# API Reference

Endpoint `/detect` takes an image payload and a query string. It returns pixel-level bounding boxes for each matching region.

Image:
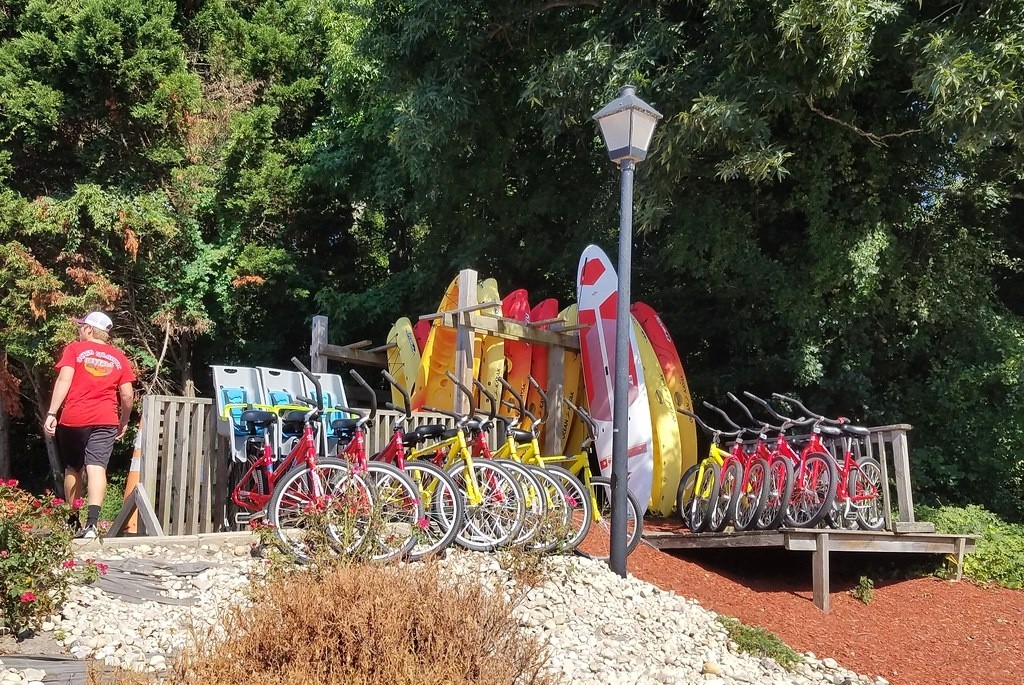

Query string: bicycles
[380,369,465,563]
[540,396,644,565]
[676,389,891,531]
[416,379,549,547]
[325,367,424,569]
[227,356,382,567]
[501,375,593,556]
[389,371,526,554]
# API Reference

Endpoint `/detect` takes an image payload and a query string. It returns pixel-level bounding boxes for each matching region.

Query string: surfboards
[385,267,591,475]
[574,244,654,520]
[630,301,698,518]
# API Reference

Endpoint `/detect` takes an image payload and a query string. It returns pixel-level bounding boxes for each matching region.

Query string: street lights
[589,83,665,580]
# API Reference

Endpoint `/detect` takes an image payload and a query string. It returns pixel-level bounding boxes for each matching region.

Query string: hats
[72,311,114,333]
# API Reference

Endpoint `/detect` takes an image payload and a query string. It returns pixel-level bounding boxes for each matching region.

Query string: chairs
[208,365,279,461]
[298,372,352,458]
[255,366,322,460]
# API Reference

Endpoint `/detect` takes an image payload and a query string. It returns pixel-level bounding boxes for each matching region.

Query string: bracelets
[47,413,56,416]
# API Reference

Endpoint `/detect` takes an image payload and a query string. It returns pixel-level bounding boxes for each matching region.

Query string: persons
[43,311,136,538]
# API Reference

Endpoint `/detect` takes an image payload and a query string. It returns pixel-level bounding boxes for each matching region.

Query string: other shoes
[81,527,97,538]
[68,524,81,538]
